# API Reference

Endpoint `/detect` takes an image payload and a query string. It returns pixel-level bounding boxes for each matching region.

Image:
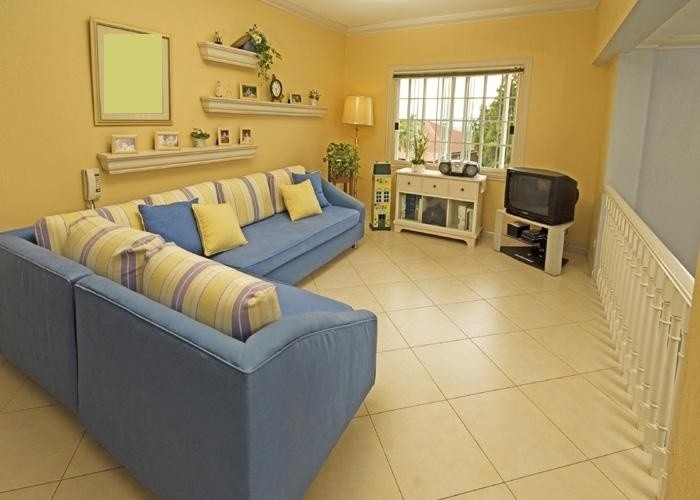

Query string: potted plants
[410,131,430,173]
[323,142,360,178]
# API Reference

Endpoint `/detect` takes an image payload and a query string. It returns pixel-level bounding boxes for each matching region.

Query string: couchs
[2,165,366,417]
[70,267,377,500]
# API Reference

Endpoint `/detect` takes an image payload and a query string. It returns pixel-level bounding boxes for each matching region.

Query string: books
[400,194,418,221]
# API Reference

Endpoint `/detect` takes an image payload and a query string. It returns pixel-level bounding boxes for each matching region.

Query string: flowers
[191,128,210,139]
[309,88,321,99]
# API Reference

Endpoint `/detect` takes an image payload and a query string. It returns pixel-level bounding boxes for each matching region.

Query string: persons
[242,131,251,142]
[246,88,254,97]
[222,131,228,143]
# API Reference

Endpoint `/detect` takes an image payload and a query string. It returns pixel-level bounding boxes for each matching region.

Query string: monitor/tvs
[504,167,579,225]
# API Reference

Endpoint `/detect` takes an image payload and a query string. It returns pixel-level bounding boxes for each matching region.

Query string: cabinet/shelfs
[491,208,575,277]
[327,172,355,199]
[97,39,329,177]
[393,167,487,248]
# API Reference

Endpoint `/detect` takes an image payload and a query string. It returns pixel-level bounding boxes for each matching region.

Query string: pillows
[138,198,204,257]
[278,179,322,222]
[291,170,330,209]
[191,200,248,257]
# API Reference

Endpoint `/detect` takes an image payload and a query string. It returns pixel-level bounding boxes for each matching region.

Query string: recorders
[439,160,480,177]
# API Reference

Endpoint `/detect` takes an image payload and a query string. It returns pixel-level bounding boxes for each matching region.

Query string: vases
[310,98,320,105]
[191,138,209,147]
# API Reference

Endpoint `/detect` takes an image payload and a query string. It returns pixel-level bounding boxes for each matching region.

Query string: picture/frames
[154,131,182,151]
[239,83,260,100]
[89,15,174,126]
[240,126,255,145]
[217,128,231,145]
[290,93,303,104]
[111,135,138,155]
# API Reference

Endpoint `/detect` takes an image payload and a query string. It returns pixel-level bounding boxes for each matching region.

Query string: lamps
[341,96,374,156]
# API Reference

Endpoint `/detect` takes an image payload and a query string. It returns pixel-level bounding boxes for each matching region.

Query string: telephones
[82,168,102,201]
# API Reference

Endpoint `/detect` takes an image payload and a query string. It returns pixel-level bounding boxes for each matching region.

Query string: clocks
[270,74,284,103]
